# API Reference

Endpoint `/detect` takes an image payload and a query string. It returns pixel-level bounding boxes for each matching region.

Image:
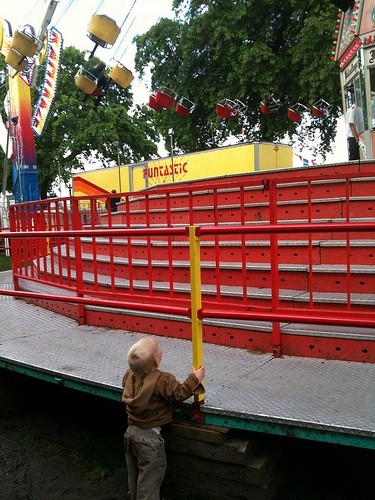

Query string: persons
[122,338,205,500]
[346,90,364,160]
[105,190,121,212]
